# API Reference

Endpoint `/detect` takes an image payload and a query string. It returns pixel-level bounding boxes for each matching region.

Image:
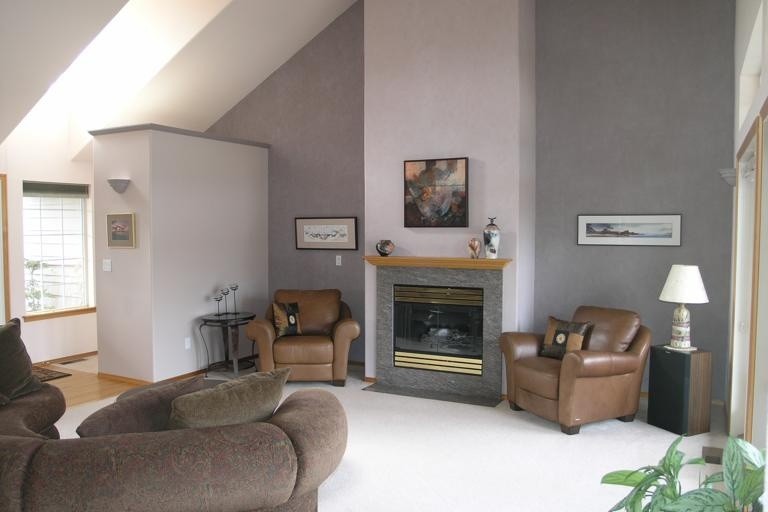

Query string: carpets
[32,364,72,383]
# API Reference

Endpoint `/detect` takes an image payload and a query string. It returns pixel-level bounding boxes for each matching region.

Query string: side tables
[201,311,257,373]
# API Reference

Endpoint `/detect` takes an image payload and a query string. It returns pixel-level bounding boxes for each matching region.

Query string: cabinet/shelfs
[648,342,714,437]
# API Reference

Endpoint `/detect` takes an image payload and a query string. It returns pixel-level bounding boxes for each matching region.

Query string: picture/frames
[576,213,682,247]
[295,216,358,250]
[105,212,137,250]
[403,158,469,228]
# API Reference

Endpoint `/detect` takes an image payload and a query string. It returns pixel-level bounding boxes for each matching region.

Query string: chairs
[245,300,361,387]
[0,383,349,512]
[499,305,653,435]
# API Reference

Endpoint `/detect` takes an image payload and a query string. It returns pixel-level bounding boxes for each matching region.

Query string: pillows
[274,289,342,336]
[169,365,293,429]
[539,317,592,360]
[271,301,303,339]
[0,318,41,409]
[75,373,206,437]
[572,306,641,352]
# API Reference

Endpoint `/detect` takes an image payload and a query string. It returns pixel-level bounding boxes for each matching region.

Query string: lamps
[658,263,710,353]
[718,168,736,187]
[211,282,240,317]
[107,178,130,194]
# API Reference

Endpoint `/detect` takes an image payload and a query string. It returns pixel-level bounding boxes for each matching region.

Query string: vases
[375,240,394,257]
[483,217,501,260]
[468,238,481,259]
